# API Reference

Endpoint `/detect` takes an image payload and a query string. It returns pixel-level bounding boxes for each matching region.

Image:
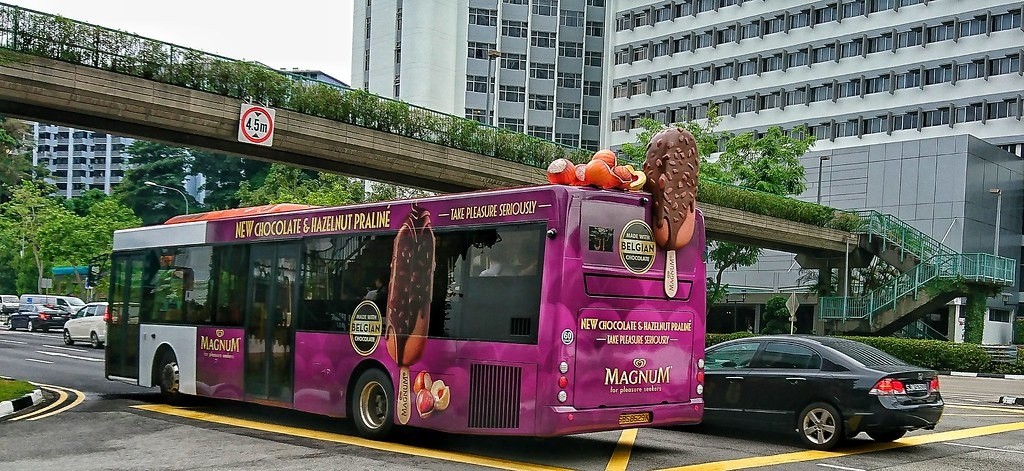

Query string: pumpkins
[546,149,633,190]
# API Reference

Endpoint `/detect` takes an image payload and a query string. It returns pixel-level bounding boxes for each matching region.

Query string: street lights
[818,156,830,205]
[144,181,189,214]
[486,49,500,126]
[989,188,1003,256]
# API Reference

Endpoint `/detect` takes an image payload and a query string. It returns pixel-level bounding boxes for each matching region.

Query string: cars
[7,303,70,332]
[677,334,945,449]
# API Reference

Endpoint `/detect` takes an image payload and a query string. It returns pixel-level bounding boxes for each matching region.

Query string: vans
[19,294,86,316]
[0,294,20,312]
[62,302,142,348]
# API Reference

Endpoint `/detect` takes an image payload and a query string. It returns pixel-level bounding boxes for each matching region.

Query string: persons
[343,262,385,302]
[479,236,538,276]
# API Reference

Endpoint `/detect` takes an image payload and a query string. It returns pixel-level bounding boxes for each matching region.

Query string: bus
[87,126,707,441]
